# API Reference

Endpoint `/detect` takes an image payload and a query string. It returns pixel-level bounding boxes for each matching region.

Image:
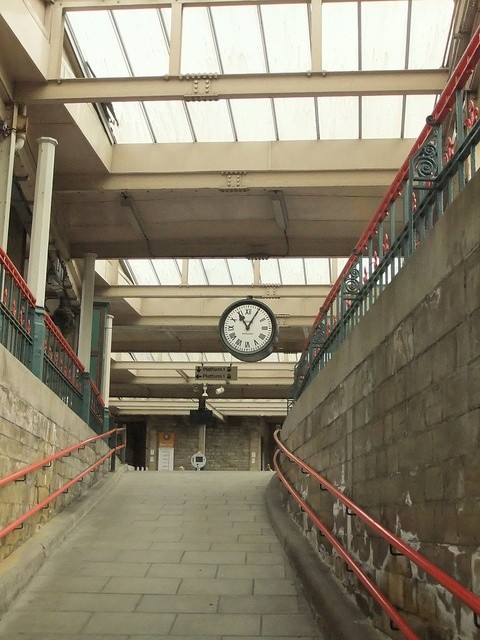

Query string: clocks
[216,298,279,362]
